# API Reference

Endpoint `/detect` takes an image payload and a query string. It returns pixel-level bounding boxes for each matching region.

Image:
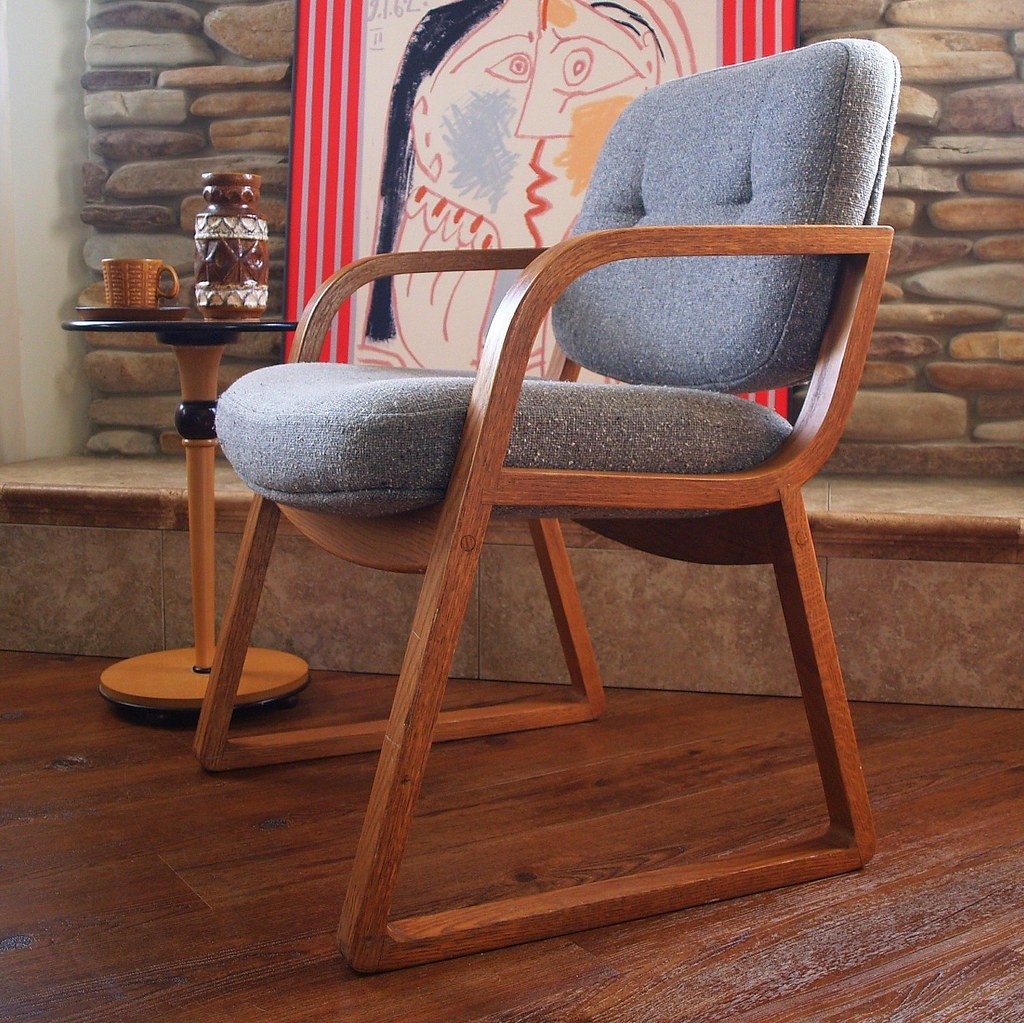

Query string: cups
[102,257,181,307]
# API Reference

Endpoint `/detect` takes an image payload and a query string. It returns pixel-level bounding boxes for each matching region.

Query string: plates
[74,305,192,321]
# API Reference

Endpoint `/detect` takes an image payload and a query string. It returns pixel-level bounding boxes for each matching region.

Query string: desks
[62,319,312,728]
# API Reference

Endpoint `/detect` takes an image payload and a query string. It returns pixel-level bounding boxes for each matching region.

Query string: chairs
[189,36,901,975]
